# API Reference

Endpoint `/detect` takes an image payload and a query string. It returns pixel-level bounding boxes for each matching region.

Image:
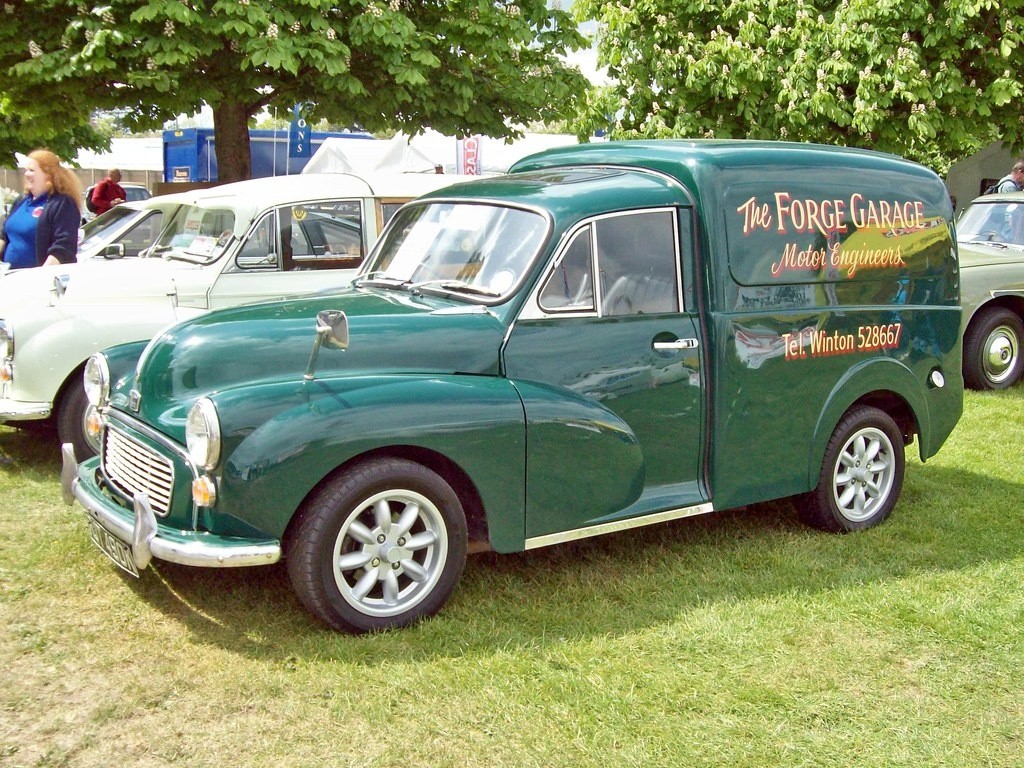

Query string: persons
[435,164,444,174]
[997,161,1024,239]
[91,168,126,219]
[0,149,82,270]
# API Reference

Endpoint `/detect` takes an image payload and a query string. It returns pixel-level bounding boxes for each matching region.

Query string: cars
[952,188,1024,392]
[1,137,967,636]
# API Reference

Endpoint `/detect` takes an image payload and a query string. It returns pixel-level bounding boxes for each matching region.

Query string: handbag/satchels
[85,180,109,212]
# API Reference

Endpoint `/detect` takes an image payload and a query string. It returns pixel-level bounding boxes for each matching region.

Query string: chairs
[600,218,677,316]
